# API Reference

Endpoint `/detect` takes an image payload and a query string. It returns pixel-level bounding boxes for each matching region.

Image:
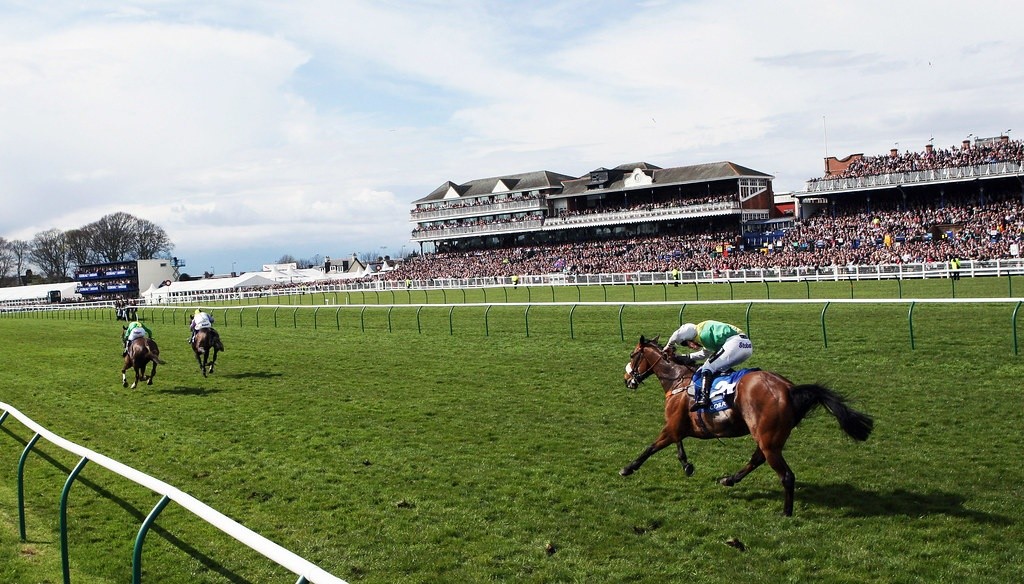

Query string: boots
[123,340,131,357]
[689,373,708,413]
[188,332,194,344]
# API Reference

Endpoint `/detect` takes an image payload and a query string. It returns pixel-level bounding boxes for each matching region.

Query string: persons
[121,322,152,357]
[389,138,1024,286]
[9,264,138,321]
[189,309,215,343]
[158,295,163,304]
[170,259,390,299]
[672,267,679,287]
[951,255,961,280]
[661,320,753,413]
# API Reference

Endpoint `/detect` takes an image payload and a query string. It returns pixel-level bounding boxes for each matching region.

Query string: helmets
[680,339,688,346]
[676,323,697,346]
[195,309,201,314]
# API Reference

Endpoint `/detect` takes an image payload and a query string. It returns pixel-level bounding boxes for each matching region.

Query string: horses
[119,324,166,389]
[617,333,876,519]
[187,314,224,379]
